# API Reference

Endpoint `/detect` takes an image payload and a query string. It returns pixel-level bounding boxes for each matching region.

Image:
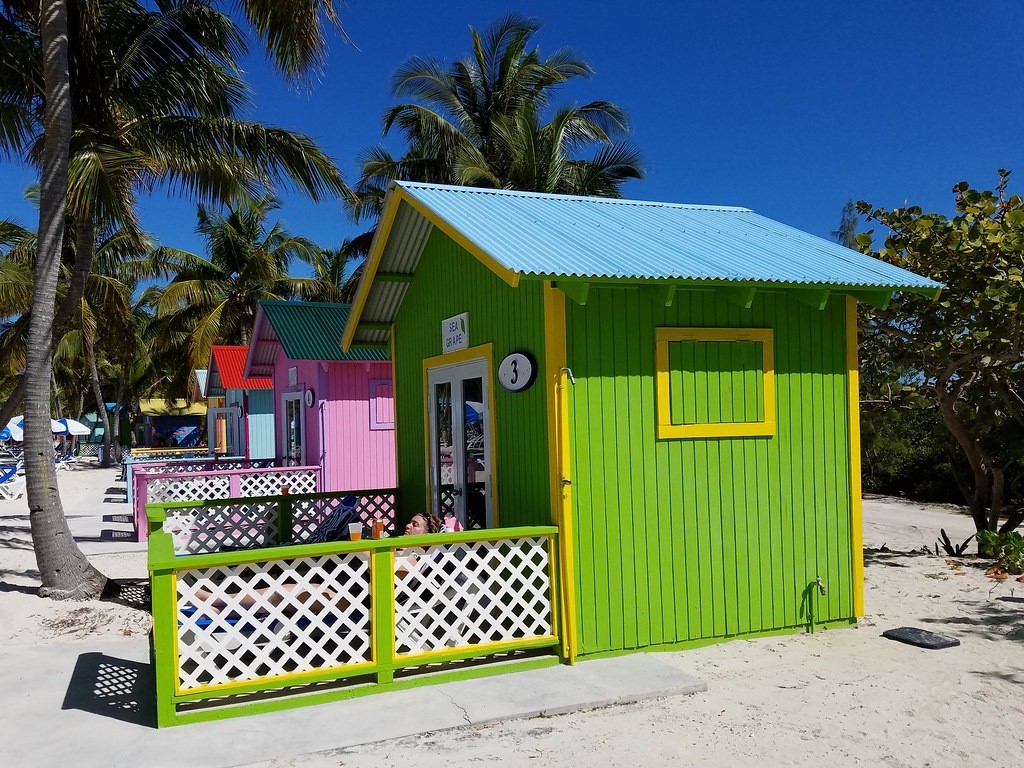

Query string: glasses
[423,512,432,533]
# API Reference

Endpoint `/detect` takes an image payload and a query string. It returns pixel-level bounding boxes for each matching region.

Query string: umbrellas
[0,415,66,441]
[53,419,91,435]
[464,401,484,424]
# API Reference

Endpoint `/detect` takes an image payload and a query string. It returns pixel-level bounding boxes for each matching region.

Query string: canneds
[372,519,384,539]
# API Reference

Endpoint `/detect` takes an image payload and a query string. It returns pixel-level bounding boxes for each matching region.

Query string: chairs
[173,509,463,633]
[0,439,81,499]
[249,460,271,486]
[221,492,363,561]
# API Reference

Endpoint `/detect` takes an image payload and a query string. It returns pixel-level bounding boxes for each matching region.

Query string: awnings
[139,398,207,416]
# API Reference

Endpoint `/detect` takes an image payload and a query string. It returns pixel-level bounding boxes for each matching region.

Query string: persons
[187,512,443,615]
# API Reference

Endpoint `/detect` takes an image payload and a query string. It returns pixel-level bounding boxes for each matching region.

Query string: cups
[281,485,289,495]
[348,522,362,541]
